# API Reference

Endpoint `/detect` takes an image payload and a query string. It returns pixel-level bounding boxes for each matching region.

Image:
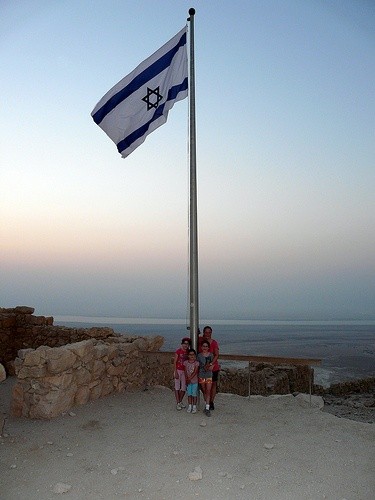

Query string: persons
[173,326,221,417]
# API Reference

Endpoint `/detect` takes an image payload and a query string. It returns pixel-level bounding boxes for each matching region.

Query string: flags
[91,21,191,158]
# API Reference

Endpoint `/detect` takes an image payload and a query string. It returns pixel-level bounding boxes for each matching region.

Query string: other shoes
[209,403,215,410]
[202,406,211,418]
[186,407,197,414]
[176,401,186,411]
